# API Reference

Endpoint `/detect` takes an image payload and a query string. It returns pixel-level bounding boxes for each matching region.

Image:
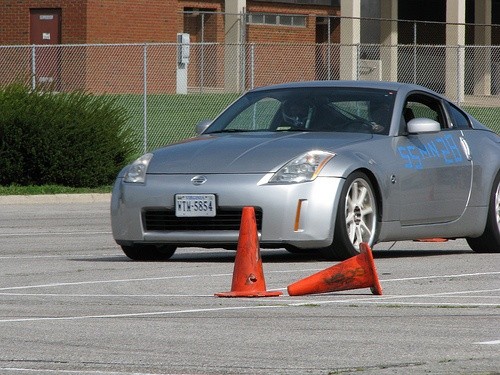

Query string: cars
[110,80,500,262]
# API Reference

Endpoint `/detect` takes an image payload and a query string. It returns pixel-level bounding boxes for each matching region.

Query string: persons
[275,98,316,130]
[370,95,407,135]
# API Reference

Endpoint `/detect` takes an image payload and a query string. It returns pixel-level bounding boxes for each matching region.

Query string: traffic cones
[286,241,383,296]
[214,206,284,298]
